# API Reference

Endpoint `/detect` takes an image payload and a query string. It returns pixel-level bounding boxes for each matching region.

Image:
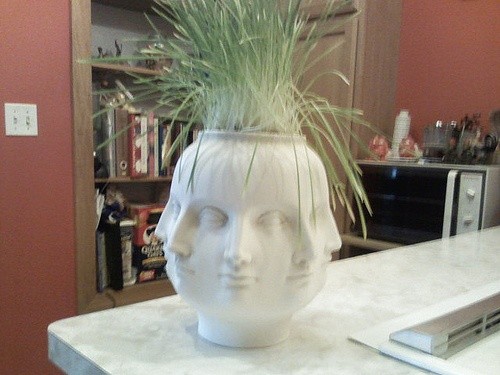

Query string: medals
[92,79,140,112]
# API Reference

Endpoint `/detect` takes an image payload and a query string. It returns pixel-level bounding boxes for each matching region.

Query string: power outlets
[4,102,39,137]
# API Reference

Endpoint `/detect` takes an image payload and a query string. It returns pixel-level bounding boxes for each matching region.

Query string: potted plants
[75,1,387,350]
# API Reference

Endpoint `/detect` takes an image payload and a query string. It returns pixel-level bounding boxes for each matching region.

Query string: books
[97,192,168,291]
[93,108,203,181]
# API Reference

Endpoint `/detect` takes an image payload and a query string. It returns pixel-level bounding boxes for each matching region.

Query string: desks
[43,222,500,375]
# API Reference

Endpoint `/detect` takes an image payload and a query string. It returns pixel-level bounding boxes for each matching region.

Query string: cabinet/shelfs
[71,0,254,310]
